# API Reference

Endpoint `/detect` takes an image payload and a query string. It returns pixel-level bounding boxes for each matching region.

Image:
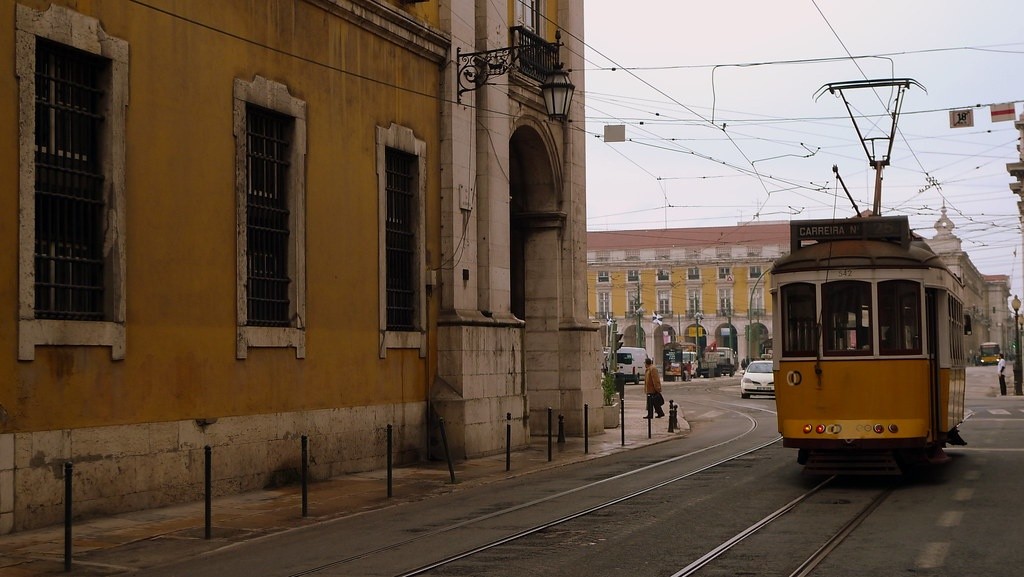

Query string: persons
[741,357,754,375]
[969,348,981,366]
[997,353,1008,397]
[686,361,692,380]
[643,358,665,419]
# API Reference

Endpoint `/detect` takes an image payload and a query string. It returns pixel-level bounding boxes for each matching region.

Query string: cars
[739,361,775,399]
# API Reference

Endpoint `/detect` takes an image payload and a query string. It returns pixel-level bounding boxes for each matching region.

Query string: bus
[979,342,1001,366]
[771,217,974,467]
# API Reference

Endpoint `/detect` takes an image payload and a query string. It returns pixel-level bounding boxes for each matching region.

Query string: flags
[652,311,663,326]
[713,343,717,352]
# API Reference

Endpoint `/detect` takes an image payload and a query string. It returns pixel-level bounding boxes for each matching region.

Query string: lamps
[457,42,575,122]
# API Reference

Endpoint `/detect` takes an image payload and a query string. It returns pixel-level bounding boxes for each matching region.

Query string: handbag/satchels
[651,392,664,406]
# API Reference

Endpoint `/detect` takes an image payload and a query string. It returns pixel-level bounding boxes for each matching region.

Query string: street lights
[1011,295,1024,396]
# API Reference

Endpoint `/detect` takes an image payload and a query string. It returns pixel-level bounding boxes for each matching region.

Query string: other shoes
[656,413,665,418]
[644,415,653,419]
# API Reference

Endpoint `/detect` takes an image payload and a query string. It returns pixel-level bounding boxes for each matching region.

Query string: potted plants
[601,372,619,428]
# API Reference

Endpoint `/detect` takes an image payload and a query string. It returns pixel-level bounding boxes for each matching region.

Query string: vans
[604,347,650,385]
